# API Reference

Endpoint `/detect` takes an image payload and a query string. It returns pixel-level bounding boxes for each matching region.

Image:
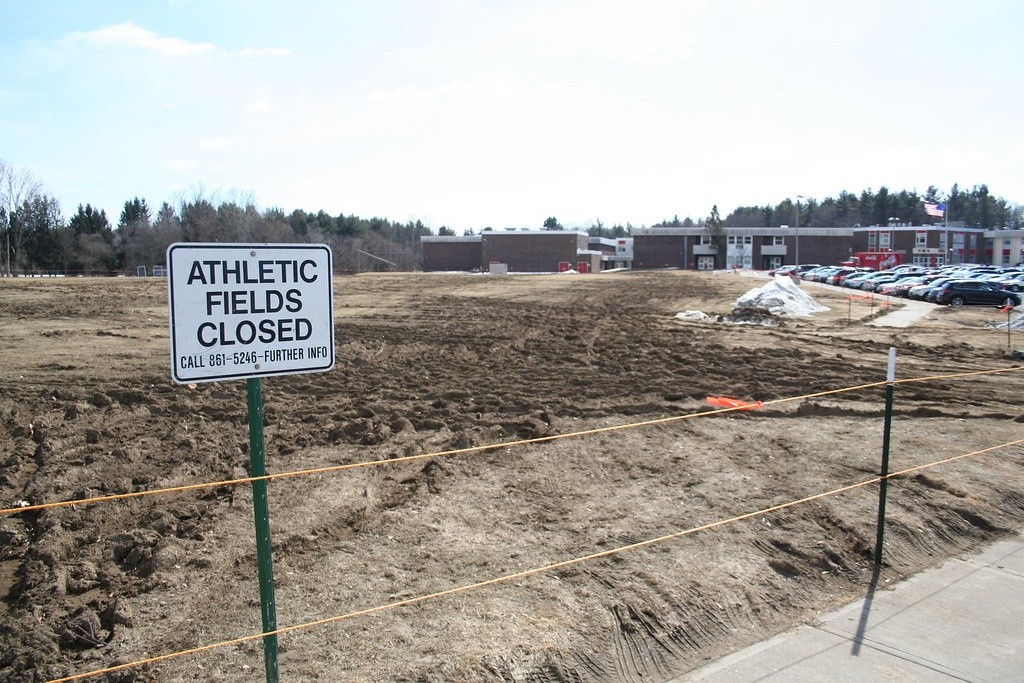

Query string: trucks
[843,252,903,271]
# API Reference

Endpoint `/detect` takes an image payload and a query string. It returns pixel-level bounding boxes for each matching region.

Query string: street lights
[795,195,804,275]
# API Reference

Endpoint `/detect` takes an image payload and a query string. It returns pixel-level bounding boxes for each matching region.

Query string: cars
[767,263,1024,305]
[936,280,1022,310]
